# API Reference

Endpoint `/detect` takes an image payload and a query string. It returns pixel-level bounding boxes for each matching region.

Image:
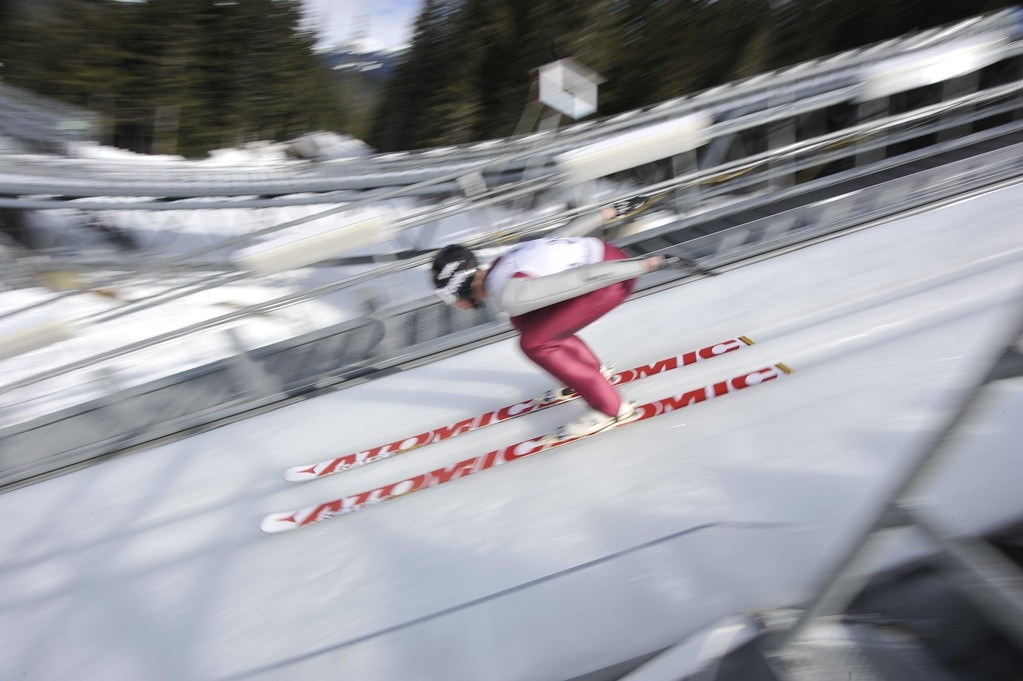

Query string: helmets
[433,244,476,303]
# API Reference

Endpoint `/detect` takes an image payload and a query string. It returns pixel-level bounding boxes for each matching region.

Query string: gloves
[648,252,695,271]
[614,195,648,215]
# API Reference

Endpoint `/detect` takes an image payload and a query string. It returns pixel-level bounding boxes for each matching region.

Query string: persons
[431,196,679,436]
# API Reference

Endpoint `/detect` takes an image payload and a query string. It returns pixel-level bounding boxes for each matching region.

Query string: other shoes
[566,401,633,440]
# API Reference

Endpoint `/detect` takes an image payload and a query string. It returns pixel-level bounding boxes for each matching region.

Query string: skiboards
[258,334,794,535]
